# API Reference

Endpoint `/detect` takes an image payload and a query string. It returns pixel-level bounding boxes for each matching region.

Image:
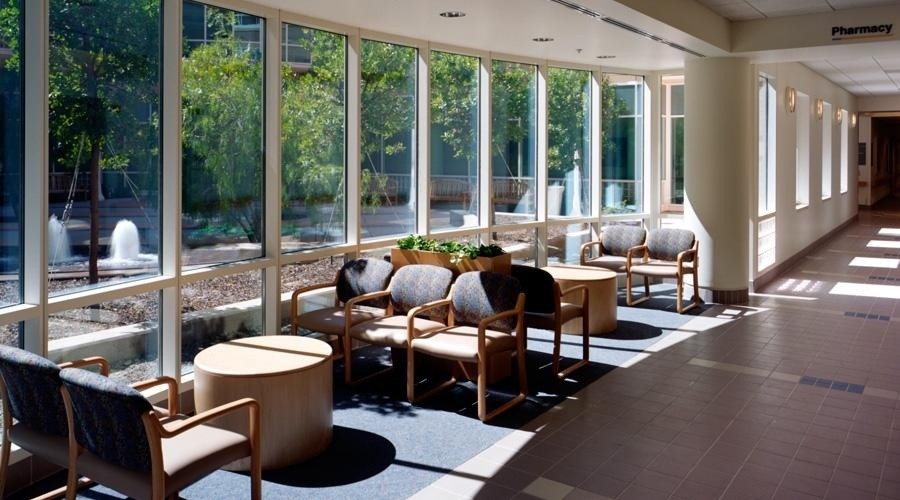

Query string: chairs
[407,270,528,424]
[59,365,261,500]
[2,342,109,499]
[291,257,394,380]
[343,264,453,399]
[626,216,700,314]
[579,217,650,305]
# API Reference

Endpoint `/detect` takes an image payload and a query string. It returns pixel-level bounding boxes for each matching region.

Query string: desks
[192,335,333,474]
[538,265,618,336]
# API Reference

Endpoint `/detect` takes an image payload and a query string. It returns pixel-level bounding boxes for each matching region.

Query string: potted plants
[392,234,512,274]
